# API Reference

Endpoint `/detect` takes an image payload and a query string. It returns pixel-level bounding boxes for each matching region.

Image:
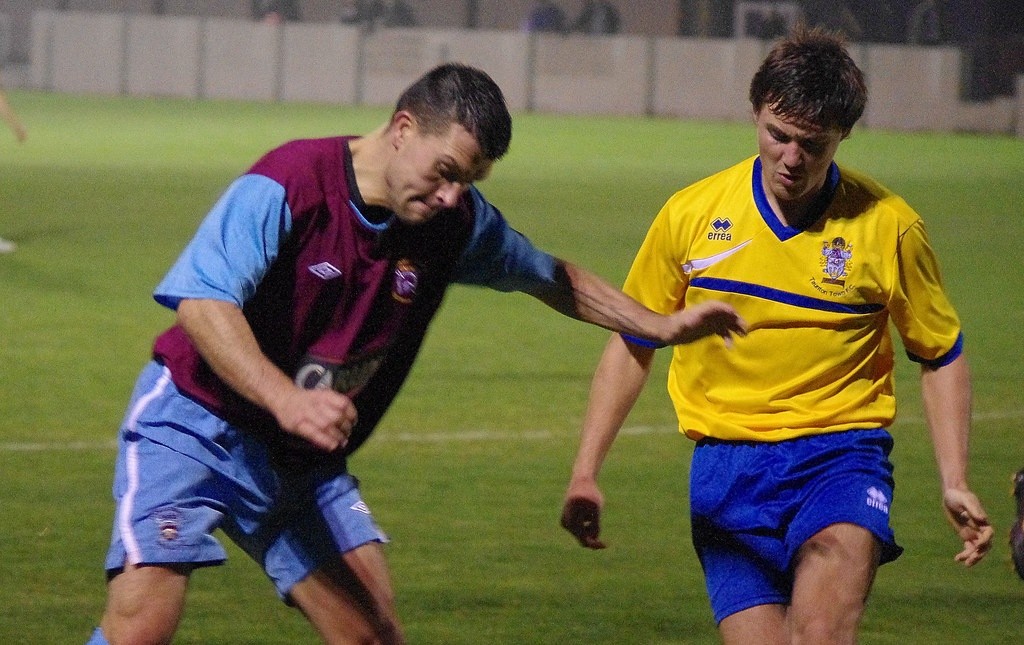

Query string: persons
[86,61,748,645]
[559,26,995,645]
